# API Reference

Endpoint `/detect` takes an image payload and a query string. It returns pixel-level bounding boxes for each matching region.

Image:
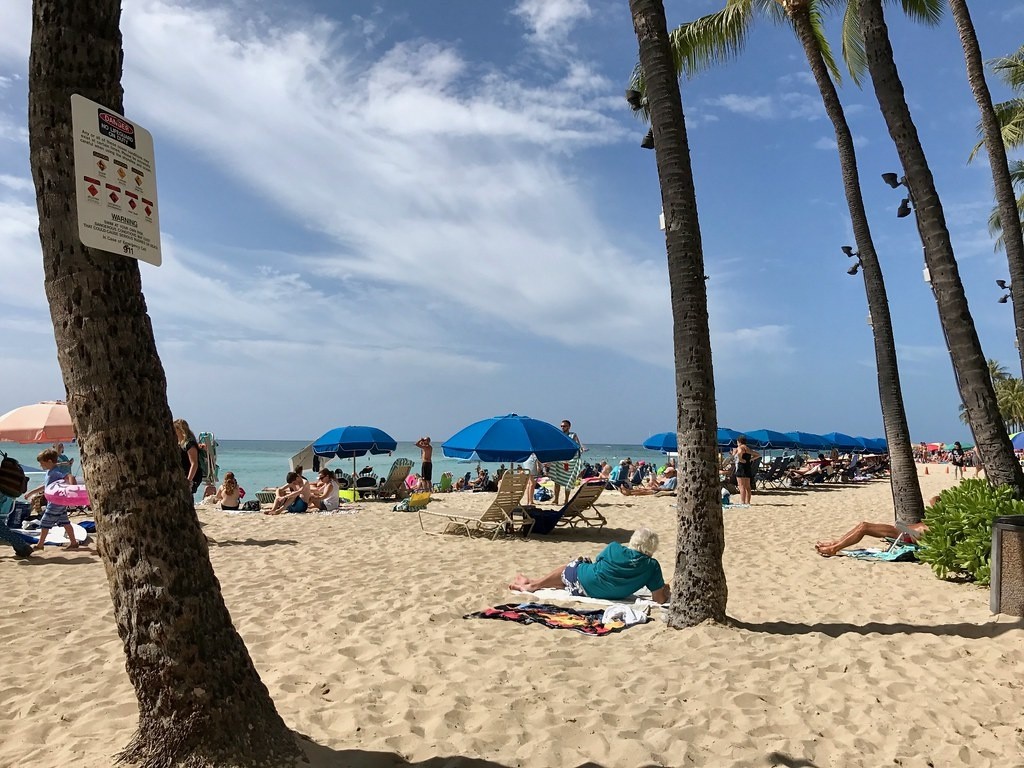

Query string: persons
[818,453,831,469]
[24,448,79,552]
[918,441,983,480]
[264,453,677,547]
[0,491,34,557]
[735,435,761,504]
[413,436,433,493]
[509,528,672,604]
[52,443,77,485]
[543,420,584,505]
[173,418,203,494]
[815,496,942,556]
[215,471,240,510]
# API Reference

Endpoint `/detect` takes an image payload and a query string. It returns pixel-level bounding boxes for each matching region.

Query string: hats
[619,460,626,464]
[664,467,675,474]
[638,461,645,465]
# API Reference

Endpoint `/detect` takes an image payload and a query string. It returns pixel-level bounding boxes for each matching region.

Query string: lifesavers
[43,476,90,506]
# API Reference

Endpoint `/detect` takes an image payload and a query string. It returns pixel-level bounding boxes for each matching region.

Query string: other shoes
[64,541,79,549]
[30,545,44,550]
[13,551,27,560]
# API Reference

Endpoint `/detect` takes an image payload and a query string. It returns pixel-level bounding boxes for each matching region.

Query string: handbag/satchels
[534,486,553,502]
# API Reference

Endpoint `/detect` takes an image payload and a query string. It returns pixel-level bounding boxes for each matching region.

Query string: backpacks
[0,449,29,498]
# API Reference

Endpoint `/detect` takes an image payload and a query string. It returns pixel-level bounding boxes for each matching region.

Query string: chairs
[469,472,489,489]
[353,473,378,500]
[581,465,594,479]
[334,473,353,490]
[513,478,609,534]
[605,454,891,492]
[417,469,536,542]
[453,471,472,491]
[348,458,415,502]
[431,471,453,493]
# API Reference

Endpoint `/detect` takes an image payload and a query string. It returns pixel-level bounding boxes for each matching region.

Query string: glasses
[561,425,567,427]
[319,474,327,481]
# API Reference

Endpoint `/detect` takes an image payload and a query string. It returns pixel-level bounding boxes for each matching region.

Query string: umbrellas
[0,400,77,453]
[1008,431,1024,449]
[643,432,678,463]
[943,441,974,450]
[717,428,943,490]
[440,413,581,534]
[312,426,398,502]
